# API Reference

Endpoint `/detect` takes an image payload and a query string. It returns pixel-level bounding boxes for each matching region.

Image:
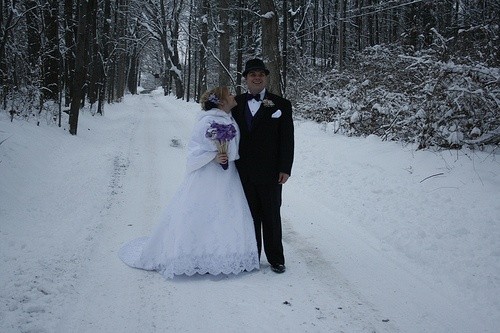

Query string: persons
[117,87,259,278]
[231,59,294,273]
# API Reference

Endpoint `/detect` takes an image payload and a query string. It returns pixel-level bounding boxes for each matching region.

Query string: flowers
[263,99,275,107]
[209,93,220,104]
[205,123,237,170]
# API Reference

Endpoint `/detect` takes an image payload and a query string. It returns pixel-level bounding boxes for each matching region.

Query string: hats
[241,58,270,76]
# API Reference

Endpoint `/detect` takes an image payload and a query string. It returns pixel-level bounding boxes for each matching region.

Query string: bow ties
[247,93,261,101]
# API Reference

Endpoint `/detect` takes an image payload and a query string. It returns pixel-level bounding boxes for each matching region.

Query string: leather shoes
[270,264,286,273]
